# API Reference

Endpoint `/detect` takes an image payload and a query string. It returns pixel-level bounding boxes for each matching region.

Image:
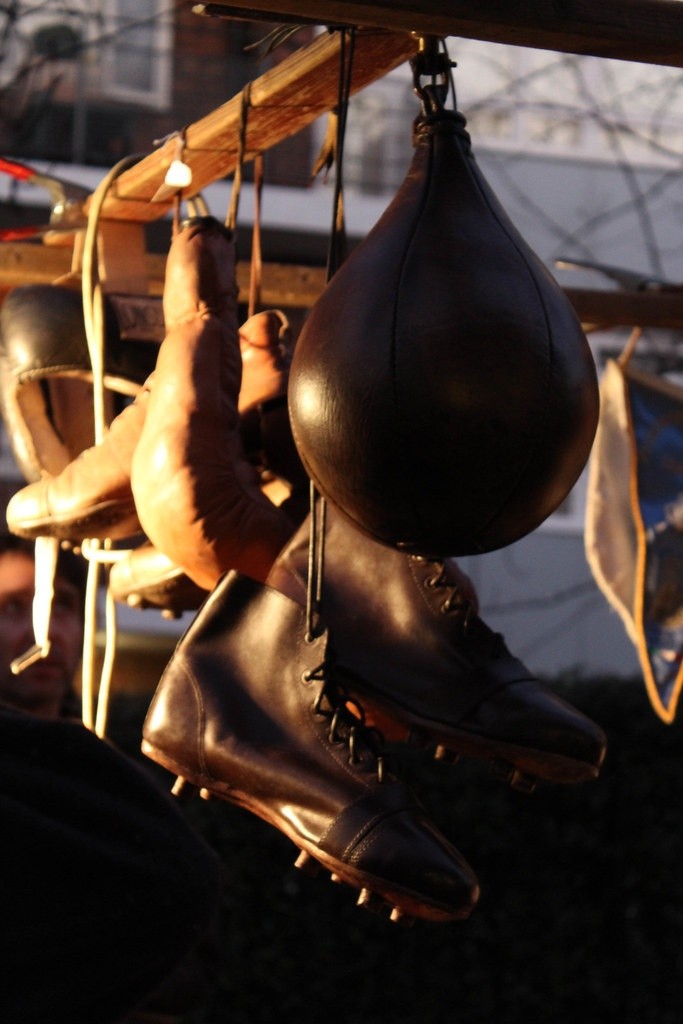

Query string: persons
[0,532,221,1024]
[642,489,683,677]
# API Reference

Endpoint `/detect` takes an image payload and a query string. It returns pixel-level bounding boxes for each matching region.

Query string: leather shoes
[261,473,606,794]
[139,570,477,923]
